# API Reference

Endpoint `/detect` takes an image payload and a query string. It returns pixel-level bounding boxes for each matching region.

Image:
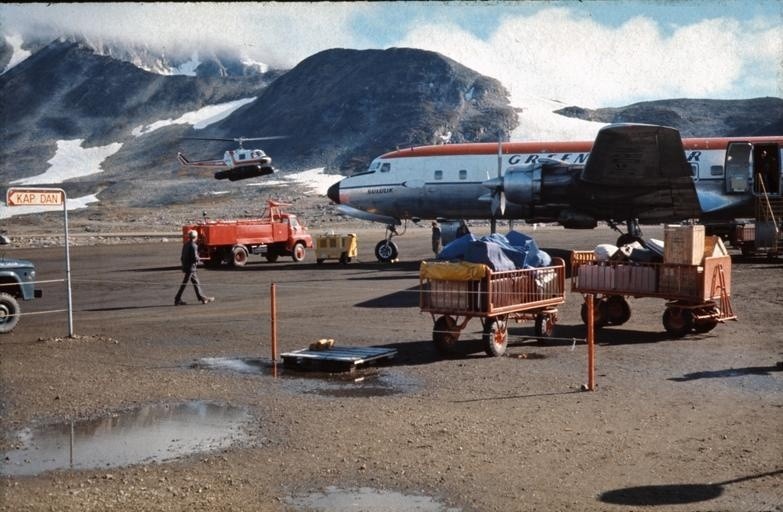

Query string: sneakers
[175,296,215,306]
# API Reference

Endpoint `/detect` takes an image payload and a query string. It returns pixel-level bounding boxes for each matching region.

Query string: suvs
[1,258,43,334]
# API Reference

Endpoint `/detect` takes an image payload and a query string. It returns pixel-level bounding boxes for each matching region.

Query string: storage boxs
[658,263,704,297]
[664,224,705,267]
[701,236,728,265]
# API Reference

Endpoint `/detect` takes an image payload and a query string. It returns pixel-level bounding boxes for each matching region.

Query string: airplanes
[327,124,783,263]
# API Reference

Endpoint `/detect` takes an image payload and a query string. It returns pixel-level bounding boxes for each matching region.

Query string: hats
[189,230,197,238]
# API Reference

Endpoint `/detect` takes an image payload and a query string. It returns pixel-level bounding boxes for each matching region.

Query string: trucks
[183,201,314,267]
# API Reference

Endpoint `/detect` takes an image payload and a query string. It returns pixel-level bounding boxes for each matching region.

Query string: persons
[173,230,214,305]
[430,221,442,256]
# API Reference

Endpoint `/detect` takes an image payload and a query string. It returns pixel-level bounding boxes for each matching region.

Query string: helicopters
[177,137,283,182]
